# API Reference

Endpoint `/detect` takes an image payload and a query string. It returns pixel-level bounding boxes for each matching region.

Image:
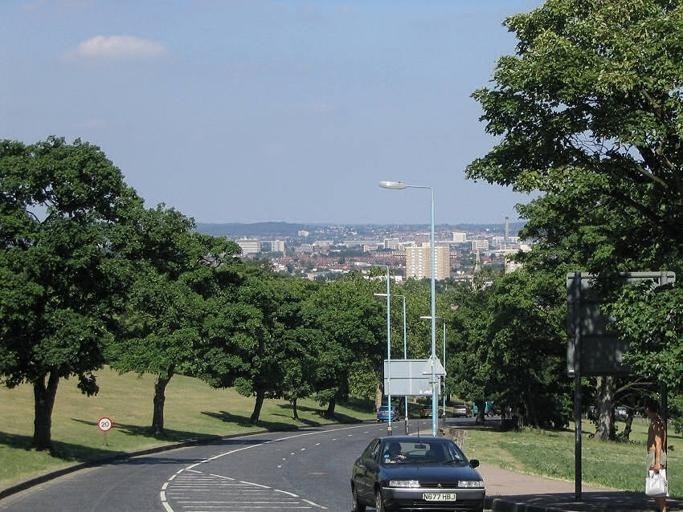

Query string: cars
[419,404,445,419]
[348,433,488,512]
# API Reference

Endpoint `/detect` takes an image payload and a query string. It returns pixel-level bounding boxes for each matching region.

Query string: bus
[472,400,493,417]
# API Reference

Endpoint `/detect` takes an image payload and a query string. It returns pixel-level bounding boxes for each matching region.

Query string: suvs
[376,405,400,422]
[452,405,472,418]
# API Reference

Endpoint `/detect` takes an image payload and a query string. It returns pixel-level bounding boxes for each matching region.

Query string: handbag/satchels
[645,477,667,496]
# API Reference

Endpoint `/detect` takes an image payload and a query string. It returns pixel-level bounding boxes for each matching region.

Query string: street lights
[418,315,447,419]
[370,292,407,435]
[358,262,393,435]
[376,179,436,437]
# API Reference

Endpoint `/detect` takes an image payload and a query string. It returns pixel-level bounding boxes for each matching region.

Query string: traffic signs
[98,416,112,431]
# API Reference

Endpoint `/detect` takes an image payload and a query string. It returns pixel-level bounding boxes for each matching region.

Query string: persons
[643,399,668,511]
[385,444,401,462]
